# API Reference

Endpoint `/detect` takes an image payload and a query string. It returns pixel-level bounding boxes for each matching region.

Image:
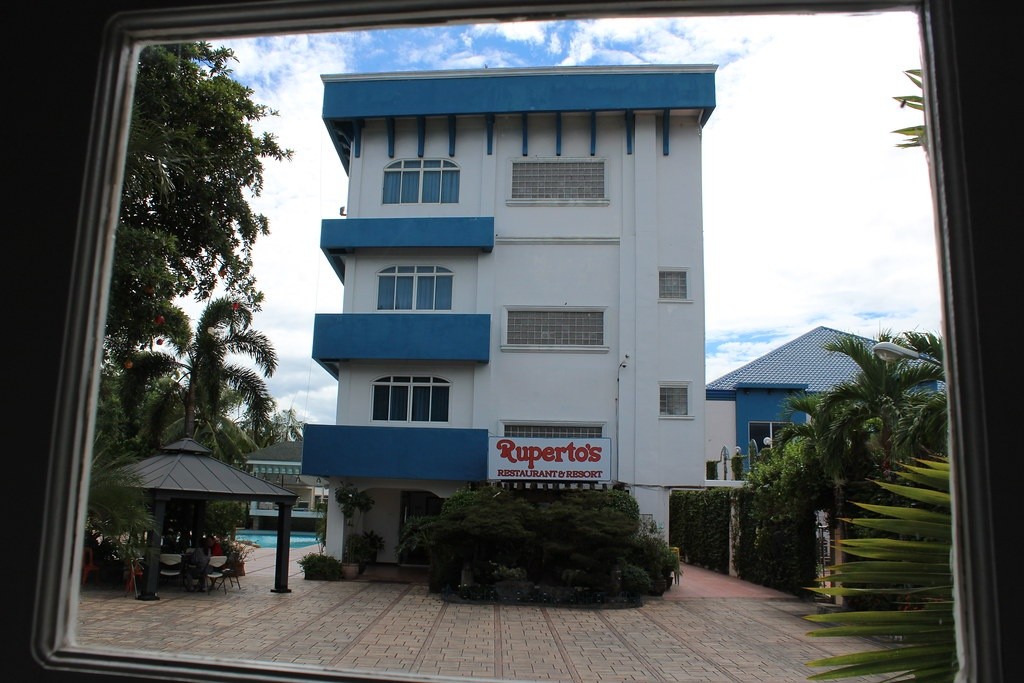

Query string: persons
[206,536,228,591]
[188,538,213,592]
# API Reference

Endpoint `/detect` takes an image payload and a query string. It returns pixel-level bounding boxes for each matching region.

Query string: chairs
[196,556,228,594]
[83,547,100,587]
[158,553,182,592]
